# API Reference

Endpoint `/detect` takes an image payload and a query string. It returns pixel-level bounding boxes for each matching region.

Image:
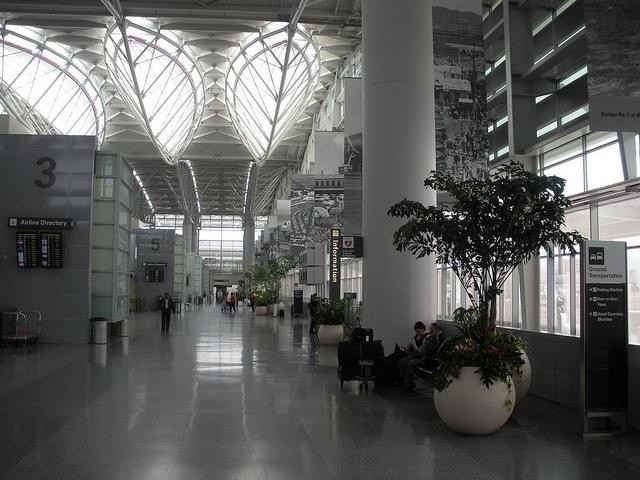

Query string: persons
[309,334,316,365]
[272,299,285,318]
[216,289,236,312]
[308,293,318,334]
[384,321,446,392]
[157,292,176,332]
[250,292,256,311]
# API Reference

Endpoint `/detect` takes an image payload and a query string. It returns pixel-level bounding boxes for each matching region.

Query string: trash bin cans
[92,317,108,344]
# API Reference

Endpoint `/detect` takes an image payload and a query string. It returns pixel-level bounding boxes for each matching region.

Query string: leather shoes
[161,328,169,331]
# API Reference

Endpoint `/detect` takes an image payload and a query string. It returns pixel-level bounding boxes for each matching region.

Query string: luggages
[338,328,387,392]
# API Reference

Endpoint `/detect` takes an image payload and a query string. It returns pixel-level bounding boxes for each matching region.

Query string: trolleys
[3,309,41,348]
[222,302,238,314]
[337,328,385,392]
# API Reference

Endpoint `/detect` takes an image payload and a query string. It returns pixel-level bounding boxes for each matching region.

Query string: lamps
[225,16,321,168]
[0,15,105,154]
[102,15,206,167]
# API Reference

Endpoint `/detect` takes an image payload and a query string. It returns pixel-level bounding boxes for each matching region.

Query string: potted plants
[243,254,296,317]
[311,296,351,346]
[390,158,587,436]
